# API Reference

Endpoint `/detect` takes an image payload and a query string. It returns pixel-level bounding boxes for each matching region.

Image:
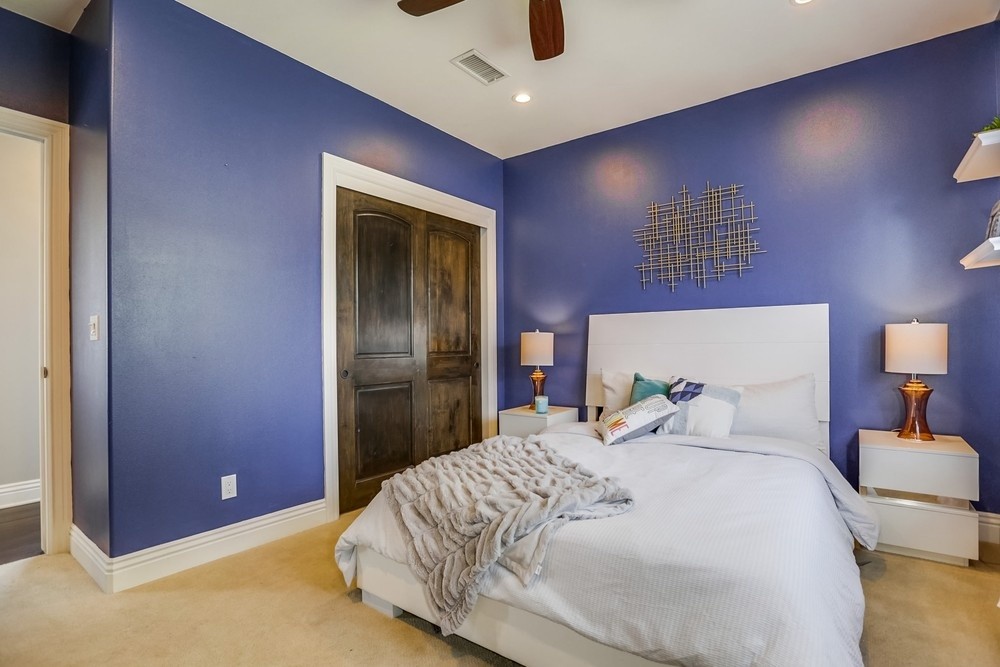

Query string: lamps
[883,316,952,443]
[520,328,555,409]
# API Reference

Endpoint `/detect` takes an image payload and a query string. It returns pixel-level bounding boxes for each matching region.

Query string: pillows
[728,372,828,451]
[593,392,681,445]
[597,368,633,422]
[631,372,669,404]
[656,376,742,436]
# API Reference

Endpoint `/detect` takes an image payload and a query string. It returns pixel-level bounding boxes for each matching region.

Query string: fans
[396,0,565,63]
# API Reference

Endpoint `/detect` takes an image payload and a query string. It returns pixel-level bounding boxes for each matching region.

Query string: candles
[535,395,548,414]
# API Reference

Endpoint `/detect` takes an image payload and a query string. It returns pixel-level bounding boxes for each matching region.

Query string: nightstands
[498,404,580,440]
[854,422,982,570]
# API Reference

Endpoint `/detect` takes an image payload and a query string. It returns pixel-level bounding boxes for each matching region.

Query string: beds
[334,302,880,667]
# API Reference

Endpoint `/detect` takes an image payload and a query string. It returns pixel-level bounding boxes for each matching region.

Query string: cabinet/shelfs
[953,129,1000,272]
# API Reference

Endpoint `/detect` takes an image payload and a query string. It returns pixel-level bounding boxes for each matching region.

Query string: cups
[535,396,548,414]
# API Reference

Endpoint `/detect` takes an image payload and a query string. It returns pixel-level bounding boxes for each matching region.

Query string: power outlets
[221,474,239,499]
